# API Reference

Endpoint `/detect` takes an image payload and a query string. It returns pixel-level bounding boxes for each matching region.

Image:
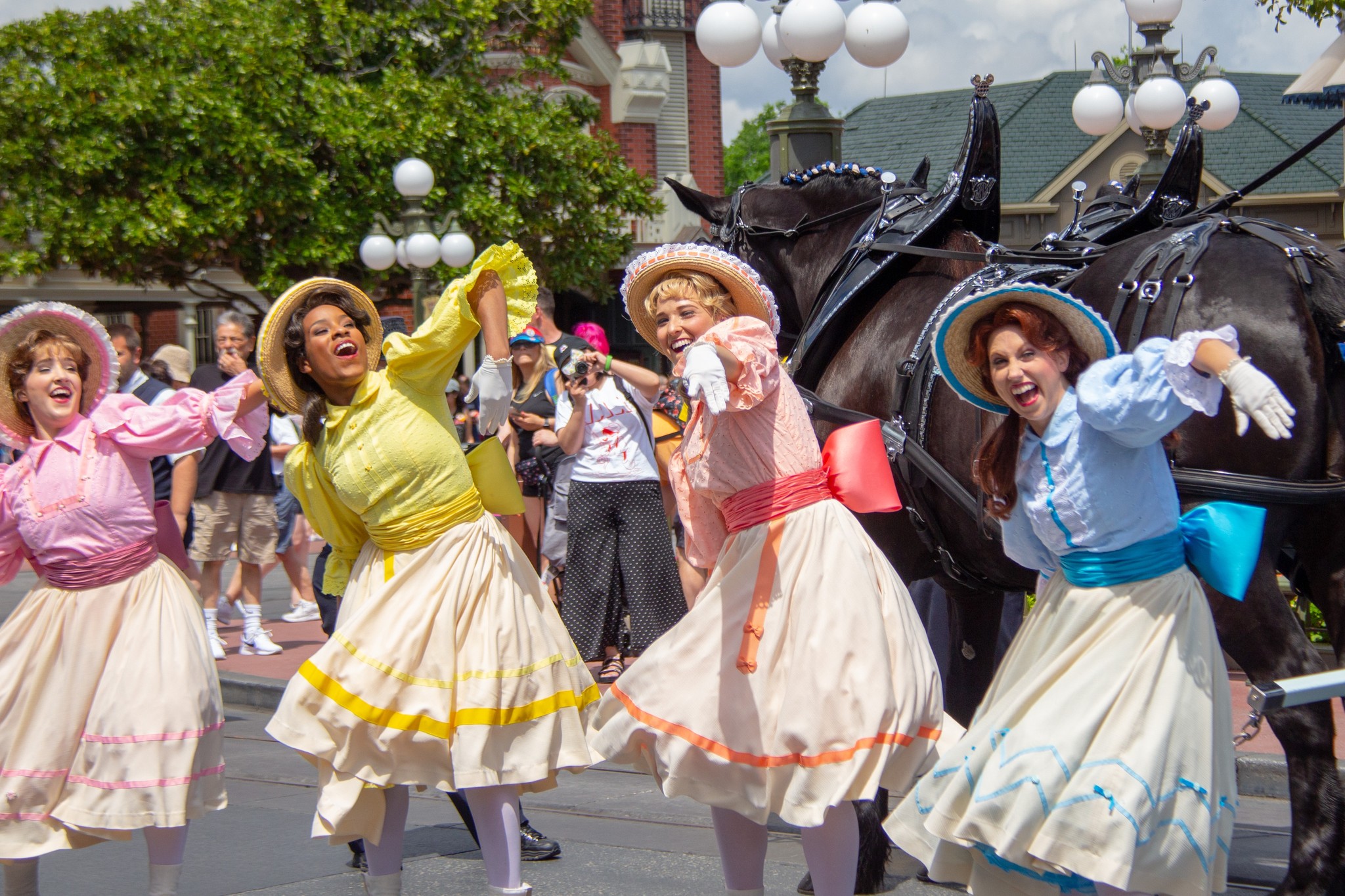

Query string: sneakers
[521,824,561,860]
[281,598,322,622]
[208,627,227,661]
[239,628,284,655]
[218,597,233,626]
[351,852,404,871]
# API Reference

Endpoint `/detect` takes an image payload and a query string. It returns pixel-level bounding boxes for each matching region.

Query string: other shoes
[290,603,300,612]
[309,534,324,542]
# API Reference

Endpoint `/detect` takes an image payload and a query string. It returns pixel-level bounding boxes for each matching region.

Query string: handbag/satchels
[514,457,549,498]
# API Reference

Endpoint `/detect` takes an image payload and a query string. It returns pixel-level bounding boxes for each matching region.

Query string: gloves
[464,355,516,436]
[1217,356,1297,439]
[683,340,729,416]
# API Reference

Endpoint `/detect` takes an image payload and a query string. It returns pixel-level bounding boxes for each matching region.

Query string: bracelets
[605,355,613,371]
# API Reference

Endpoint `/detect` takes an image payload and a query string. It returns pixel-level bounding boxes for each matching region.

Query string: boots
[487,881,532,896]
[359,871,402,896]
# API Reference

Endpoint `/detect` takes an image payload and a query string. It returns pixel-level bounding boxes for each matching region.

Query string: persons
[585,243,942,896]
[256,240,602,896]
[106,284,706,684]
[0,301,274,896]
[312,543,562,872]
[882,281,1295,896]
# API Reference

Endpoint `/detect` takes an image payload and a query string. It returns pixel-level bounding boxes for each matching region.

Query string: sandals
[598,653,625,684]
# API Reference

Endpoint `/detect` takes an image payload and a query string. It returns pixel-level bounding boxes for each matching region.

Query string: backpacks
[540,454,576,568]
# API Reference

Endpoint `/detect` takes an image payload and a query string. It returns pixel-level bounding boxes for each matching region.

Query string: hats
[553,336,595,375]
[150,344,192,384]
[930,282,1121,416]
[256,277,384,417]
[1,301,121,452]
[619,244,781,355]
[509,325,544,345]
[445,378,461,393]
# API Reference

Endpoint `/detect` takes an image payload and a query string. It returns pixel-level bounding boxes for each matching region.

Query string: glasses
[510,341,539,351]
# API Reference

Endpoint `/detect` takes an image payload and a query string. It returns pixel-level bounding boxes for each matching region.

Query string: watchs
[543,418,550,429]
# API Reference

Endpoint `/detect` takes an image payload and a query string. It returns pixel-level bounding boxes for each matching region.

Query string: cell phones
[510,412,522,417]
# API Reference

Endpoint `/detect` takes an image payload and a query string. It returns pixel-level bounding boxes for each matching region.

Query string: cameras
[574,361,588,378]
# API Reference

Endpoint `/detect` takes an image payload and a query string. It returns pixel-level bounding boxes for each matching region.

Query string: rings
[221,362,225,366]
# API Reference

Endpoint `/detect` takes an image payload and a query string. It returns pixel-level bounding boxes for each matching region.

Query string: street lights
[359,158,476,332]
[695,1,911,185]
[1071,0,1240,210]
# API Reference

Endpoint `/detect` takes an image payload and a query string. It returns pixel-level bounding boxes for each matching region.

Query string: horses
[1083,184,1200,231]
[663,161,1345,896]
